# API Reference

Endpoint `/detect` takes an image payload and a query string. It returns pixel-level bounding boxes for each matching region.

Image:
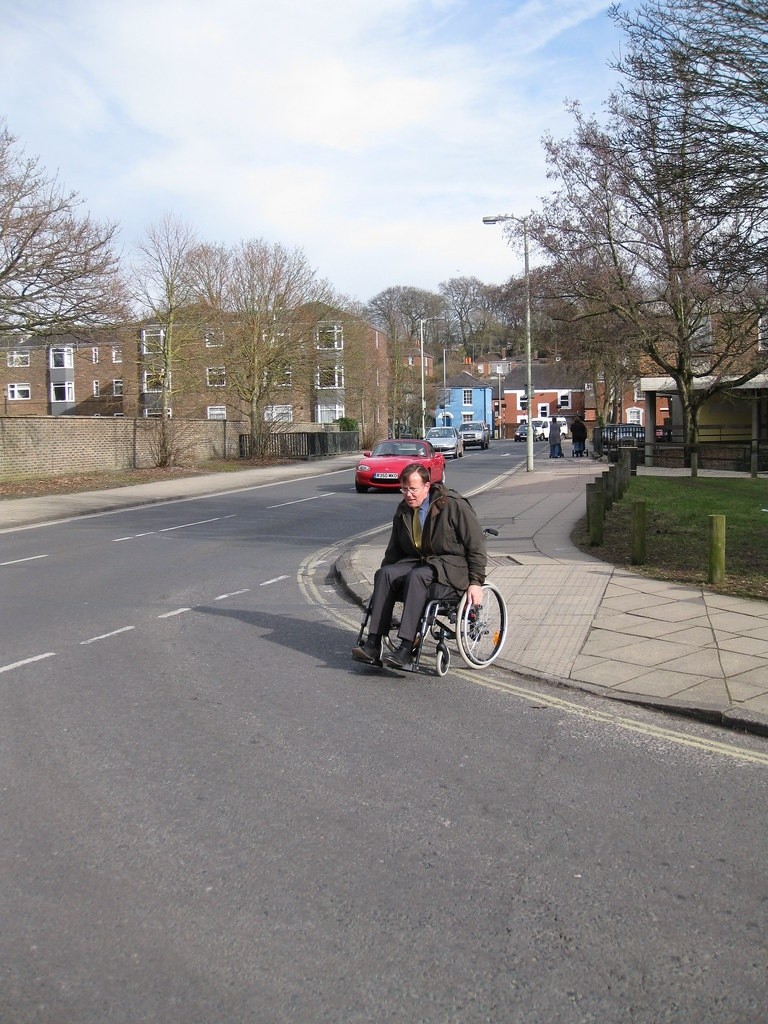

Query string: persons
[549,417,561,458]
[570,417,587,457]
[351,463,488,668]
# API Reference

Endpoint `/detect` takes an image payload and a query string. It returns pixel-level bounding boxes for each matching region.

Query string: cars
[459,420,490,450]
[355,439,447,494]
[601,427,605,440]
[602,424,623,442]
[614,423,645,443]
[424,426,465,460]
[514,424,539,442]
[656,429,672,438]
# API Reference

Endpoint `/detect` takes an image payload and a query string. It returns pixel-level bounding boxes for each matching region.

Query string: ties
[412,507,422,550]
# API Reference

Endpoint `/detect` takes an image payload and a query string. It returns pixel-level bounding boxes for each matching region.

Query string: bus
[531,417,569,442]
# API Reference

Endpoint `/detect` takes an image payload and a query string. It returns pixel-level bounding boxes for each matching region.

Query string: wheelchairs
[356,528,508,677]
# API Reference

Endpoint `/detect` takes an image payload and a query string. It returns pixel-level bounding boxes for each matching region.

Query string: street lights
[483,215,534,472]
[497,364,502,440]
[421,317,446,440]
[443,347,460,428]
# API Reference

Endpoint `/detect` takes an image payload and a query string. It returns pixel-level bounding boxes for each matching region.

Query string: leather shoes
[383,646,413,667]
[352,639,381,660]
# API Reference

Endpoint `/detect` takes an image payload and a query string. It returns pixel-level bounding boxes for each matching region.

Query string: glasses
[400,483,425,493]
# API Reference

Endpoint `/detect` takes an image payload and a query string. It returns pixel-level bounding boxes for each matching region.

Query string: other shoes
[580,455,583,457]
[576,455,578,457]
